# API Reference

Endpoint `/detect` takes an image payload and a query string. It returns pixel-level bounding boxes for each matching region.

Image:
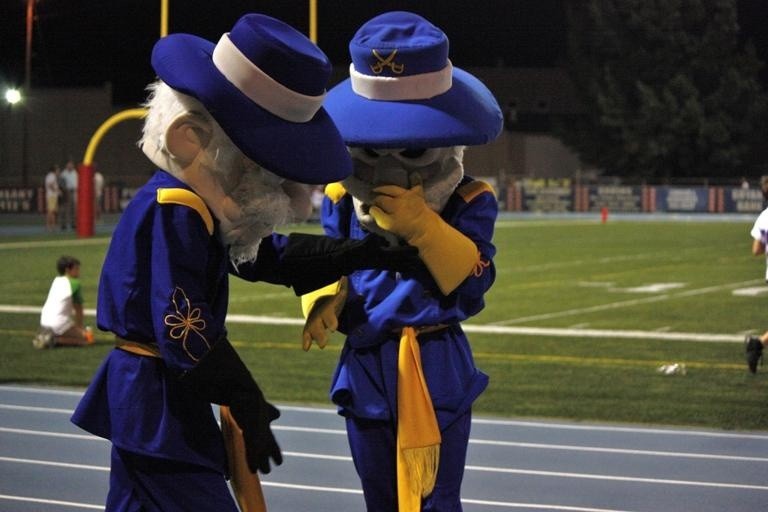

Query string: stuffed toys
[294,9,508,512]
[66,13,402,512]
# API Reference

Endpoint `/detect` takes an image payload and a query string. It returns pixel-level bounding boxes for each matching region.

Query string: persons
[741,176,749,189]
[743,174,768,374]
[44,164,64,233]
[94,171,104,220]
[58,160,78,230]
[32,255,89,350]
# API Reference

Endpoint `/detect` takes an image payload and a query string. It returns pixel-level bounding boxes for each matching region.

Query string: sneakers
[745,336,764,374]
[33,333,55,350]
